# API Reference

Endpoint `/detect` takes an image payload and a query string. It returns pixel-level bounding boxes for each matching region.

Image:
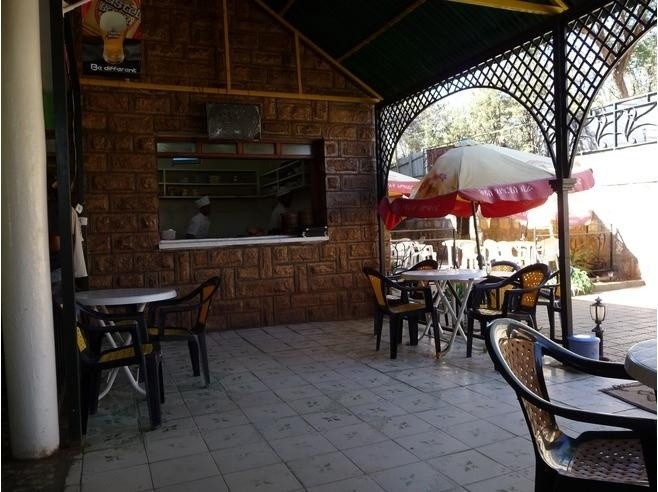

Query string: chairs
[486,315,657,488]
[71,275,220,437]
[360,259,562,370]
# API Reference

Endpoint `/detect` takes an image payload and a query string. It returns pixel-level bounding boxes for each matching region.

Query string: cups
[100,16,126,64]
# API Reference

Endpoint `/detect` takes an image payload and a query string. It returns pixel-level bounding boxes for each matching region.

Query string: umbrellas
[388,168,426,200]
[379,143,597,272]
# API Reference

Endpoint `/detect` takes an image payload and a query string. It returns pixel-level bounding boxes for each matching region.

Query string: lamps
[589,297,608,362]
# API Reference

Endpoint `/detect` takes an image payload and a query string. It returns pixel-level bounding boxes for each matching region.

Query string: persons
[184,195,211,239]
[265,186,308,235]
[46,183,89,318]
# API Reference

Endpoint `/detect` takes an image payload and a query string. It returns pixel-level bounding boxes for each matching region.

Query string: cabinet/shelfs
[156,159,312,203]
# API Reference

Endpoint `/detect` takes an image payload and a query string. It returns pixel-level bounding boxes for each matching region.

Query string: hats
[275,186,290,197]
[195,196,210,209]
